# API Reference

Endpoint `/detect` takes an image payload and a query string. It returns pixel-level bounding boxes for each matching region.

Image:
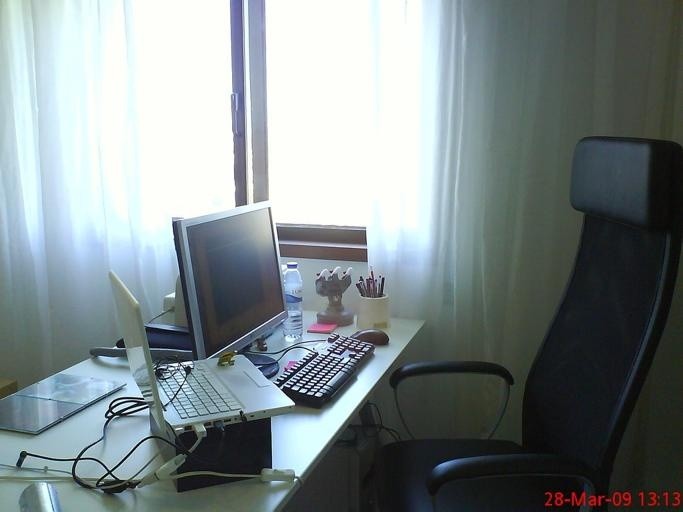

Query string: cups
[358,295,389,330]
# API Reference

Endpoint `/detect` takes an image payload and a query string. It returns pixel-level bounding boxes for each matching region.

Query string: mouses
[349,328,390,345]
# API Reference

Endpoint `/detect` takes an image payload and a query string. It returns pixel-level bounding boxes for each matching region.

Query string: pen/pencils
[356,265,385,298]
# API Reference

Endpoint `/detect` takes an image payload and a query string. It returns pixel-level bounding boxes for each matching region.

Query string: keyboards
[272,330,376,408]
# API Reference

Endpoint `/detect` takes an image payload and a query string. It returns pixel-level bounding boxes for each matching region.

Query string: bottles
[283,260,302,341]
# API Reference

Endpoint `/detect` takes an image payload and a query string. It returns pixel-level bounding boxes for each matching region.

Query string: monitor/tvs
[172,199,289,379]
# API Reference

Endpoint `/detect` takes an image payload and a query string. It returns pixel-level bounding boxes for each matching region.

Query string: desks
[0,312,426,512]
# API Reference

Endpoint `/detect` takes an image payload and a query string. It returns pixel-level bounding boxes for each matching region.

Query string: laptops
[108,268,296,436]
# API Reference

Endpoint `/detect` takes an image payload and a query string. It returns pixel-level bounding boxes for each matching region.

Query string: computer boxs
[284,429,401,512]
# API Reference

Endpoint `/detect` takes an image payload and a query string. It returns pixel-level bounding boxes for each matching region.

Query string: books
[0,370,127,437]
[306,322,339,334]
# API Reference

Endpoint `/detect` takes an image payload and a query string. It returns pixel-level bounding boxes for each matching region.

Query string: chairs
[372,135,683,512]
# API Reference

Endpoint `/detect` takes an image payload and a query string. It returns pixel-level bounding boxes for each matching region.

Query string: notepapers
[307,323,337,334]
